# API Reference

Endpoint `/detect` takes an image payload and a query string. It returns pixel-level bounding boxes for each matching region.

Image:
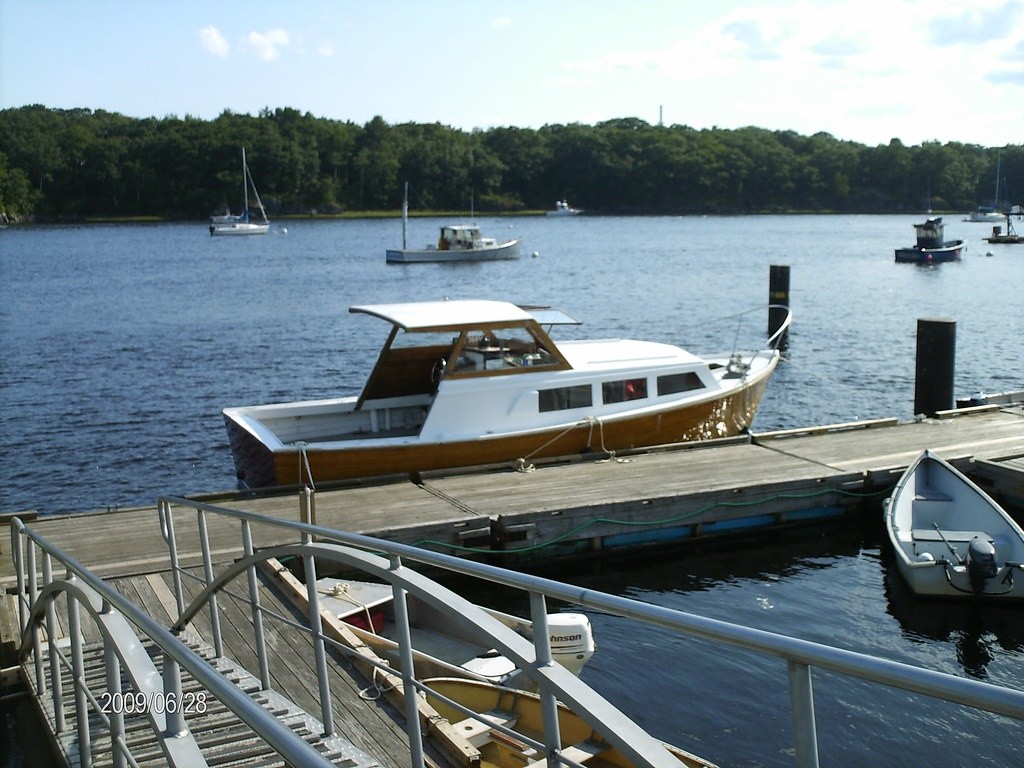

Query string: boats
[894,216,966,262]
[210,210,244,223]
[221,299,793,490]
[884,447,1024,601]
[545,198,584,216]
[982,216,1020,242]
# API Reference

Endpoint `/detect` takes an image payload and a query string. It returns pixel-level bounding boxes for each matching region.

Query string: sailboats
[385,180,521,263]
[210,145,271,236]
[963,148,1008,223]
